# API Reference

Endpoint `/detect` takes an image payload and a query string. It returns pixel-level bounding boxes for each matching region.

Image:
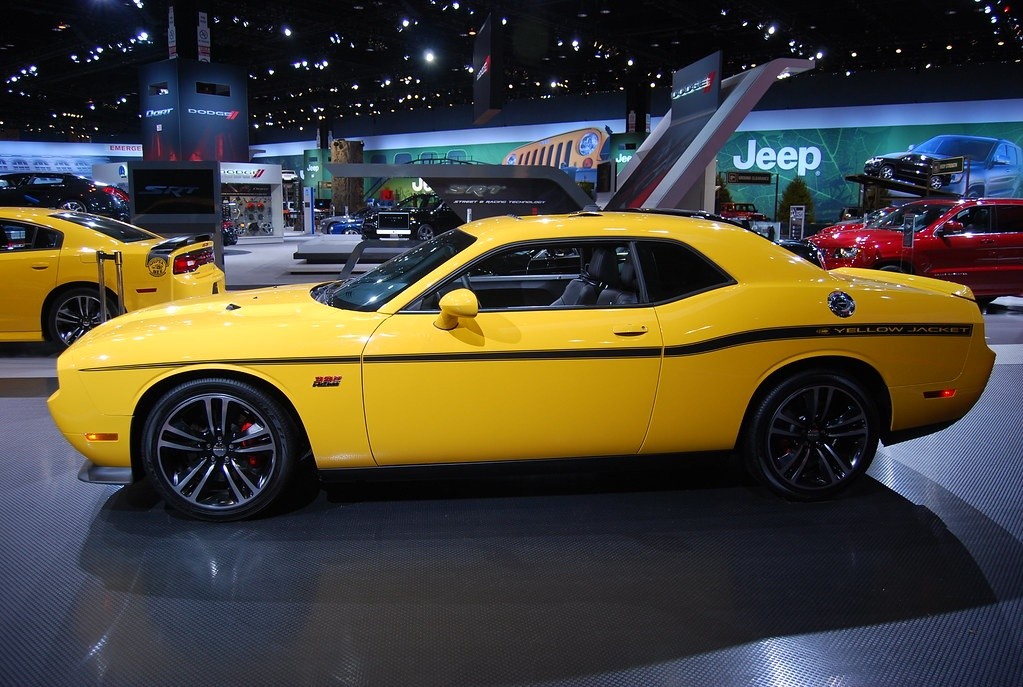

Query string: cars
[47,205,999,521]
[0,205,227,354]
[196,216,238,246]
[362,194,464,241]
[282,201,325,227]
[467,247,630,275]
[319,204,395,234]
[800,197,1023,301]
[0,172,129,226]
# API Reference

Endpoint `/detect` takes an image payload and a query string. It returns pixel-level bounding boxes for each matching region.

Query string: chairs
[550,248,609,307]
[598,254,638,304]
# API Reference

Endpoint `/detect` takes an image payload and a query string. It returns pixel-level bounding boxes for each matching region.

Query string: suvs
[500,128,607,192]
[862,133,1023,200]
[865,152,952,190]
[720,203,770,223]
